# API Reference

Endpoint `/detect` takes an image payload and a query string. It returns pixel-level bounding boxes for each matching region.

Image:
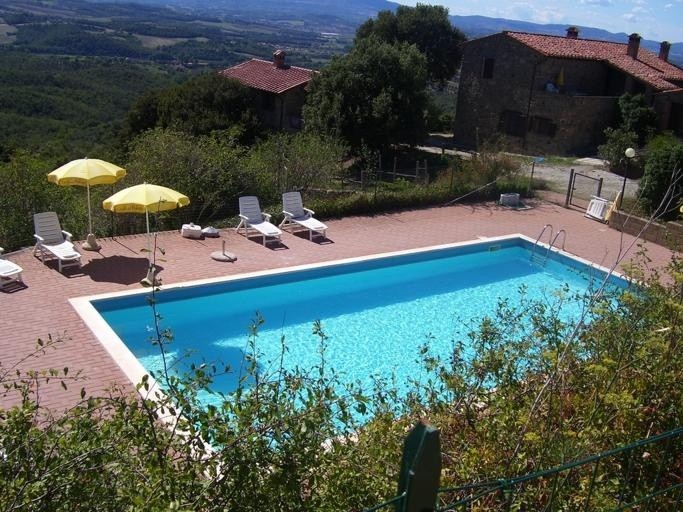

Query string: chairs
[0,247,23,290]
[33,212,81,272]
[279,191,328,241]
[236,196,282,247]
[584,195,609,223]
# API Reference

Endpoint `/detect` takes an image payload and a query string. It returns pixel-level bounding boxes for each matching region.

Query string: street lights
[620,148,635,206]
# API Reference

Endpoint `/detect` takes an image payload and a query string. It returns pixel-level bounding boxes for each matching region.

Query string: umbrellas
[101,181,192,267]
[46,156,129,234]
